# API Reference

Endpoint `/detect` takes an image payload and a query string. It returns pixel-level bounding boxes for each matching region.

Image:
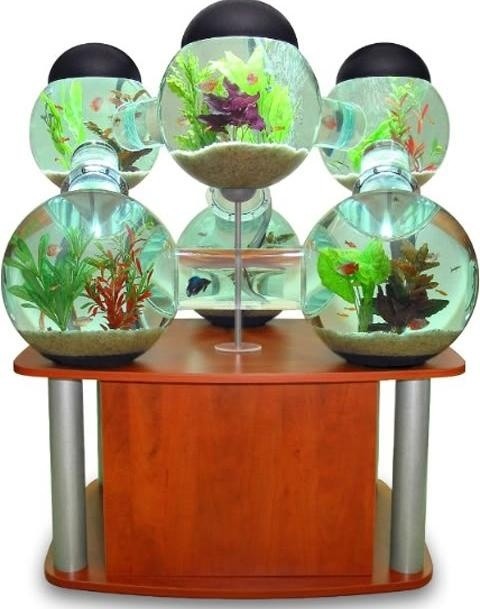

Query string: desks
[12,318,466,600]
